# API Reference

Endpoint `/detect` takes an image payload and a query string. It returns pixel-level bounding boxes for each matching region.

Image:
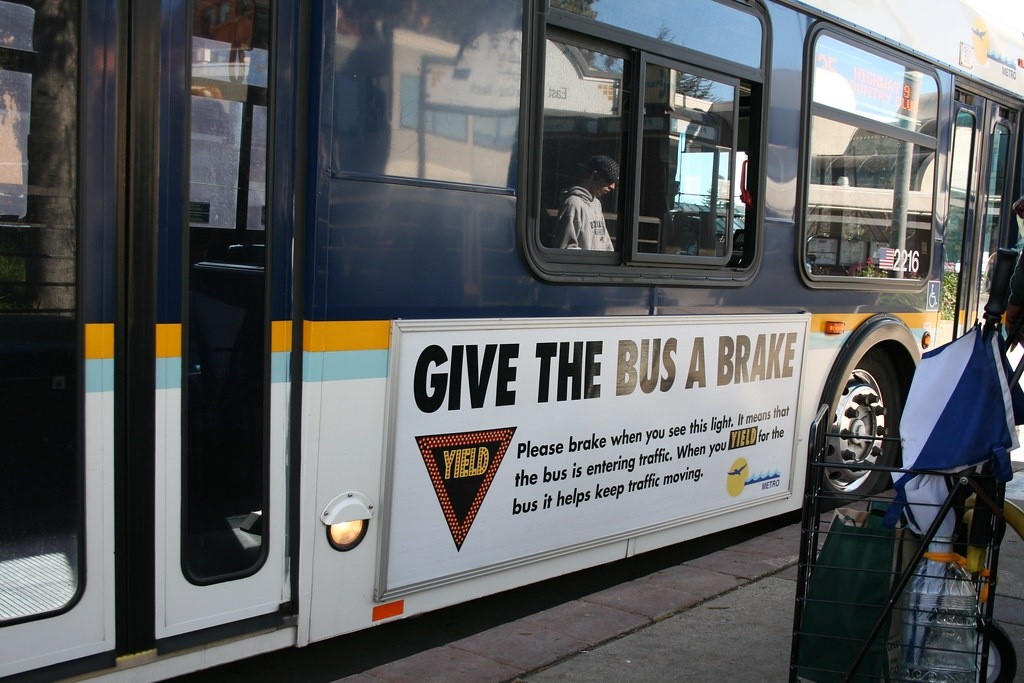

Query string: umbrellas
[881,248,1021,665]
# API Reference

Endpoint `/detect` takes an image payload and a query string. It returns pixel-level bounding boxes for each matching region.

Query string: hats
[592,155,620,182]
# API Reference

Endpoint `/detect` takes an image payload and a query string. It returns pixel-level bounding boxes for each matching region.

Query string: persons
[1004,251,1024,345]
[552,155,620,251]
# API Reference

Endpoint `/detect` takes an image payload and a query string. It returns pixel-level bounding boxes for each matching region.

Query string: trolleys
[787,312,1024,683]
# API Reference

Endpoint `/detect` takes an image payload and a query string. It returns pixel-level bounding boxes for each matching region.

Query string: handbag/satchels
[795,507,918,683]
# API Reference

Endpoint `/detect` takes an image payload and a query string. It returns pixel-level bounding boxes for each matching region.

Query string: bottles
[229,41,246,83]
[897,552,978,683]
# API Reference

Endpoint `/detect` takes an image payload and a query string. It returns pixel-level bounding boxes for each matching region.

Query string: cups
[836,176,849,186]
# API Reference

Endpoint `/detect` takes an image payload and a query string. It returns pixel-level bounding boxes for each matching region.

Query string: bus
[0,0,1024,683]
